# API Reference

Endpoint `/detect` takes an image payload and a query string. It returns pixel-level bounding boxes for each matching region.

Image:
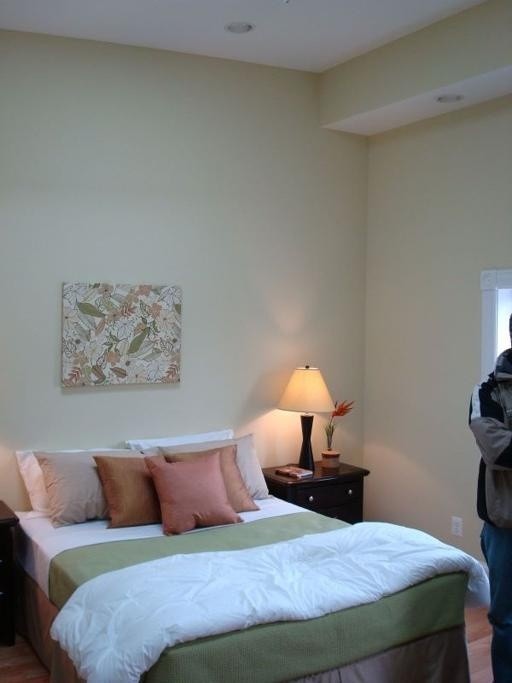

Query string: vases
[323,451,341,469]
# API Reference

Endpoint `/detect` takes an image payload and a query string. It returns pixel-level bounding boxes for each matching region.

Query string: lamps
[278,366,335,477]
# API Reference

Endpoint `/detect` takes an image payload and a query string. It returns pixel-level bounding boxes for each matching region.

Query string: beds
[9,433,491,683]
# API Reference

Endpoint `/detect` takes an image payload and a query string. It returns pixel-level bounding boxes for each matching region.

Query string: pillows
[127,431,232,449]
[14,449,49,512]
[145,453,242,535]
[166,444,260,513]
[93,454,162,528]
[148,434,268,498]
[33,451,142,529]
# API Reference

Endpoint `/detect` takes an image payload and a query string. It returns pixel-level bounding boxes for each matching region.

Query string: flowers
[325,399,351,448]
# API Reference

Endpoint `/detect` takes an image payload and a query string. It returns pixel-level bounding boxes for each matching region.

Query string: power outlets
[451,515,464,537]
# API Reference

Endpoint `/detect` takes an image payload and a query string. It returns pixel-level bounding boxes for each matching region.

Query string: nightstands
[1,499,19,649]
[263,459,369,524]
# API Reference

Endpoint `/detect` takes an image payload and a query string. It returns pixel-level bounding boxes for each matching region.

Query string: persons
[467,314,511,683]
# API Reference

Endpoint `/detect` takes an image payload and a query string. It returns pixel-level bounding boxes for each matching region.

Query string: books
[274,465,314,479]
